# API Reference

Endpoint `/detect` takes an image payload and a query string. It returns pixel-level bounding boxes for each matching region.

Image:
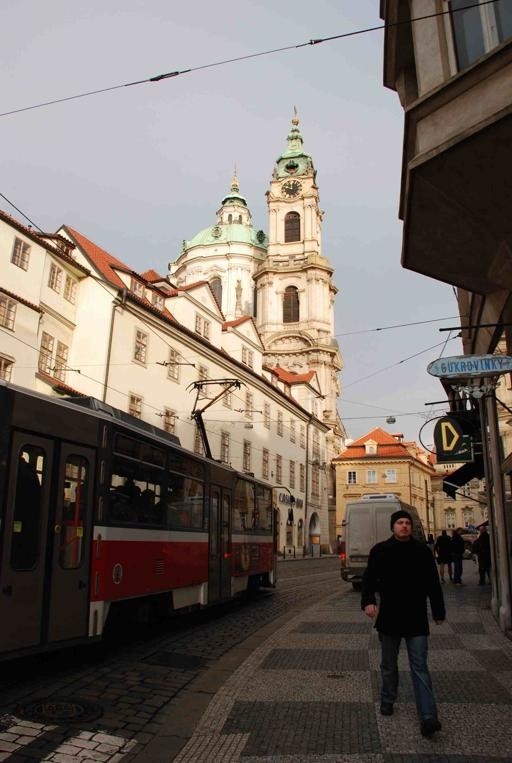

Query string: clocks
[281,180,303,199]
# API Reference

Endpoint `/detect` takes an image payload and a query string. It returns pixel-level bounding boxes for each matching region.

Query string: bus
[1,369,295,665]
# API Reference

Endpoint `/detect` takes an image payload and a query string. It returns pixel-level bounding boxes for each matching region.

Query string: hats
[390,510,414,531]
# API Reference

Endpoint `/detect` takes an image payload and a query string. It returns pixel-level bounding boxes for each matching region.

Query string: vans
[339,494,428,591]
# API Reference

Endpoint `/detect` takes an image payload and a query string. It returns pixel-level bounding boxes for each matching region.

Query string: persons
[451,527,465,585]
[360,508,447,737]
[435,529,453,584]
[426,534,435,559]
[474,525,491,586]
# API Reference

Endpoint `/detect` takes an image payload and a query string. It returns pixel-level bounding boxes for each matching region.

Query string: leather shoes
[379,700,394,716]
[420,718,442,736]
